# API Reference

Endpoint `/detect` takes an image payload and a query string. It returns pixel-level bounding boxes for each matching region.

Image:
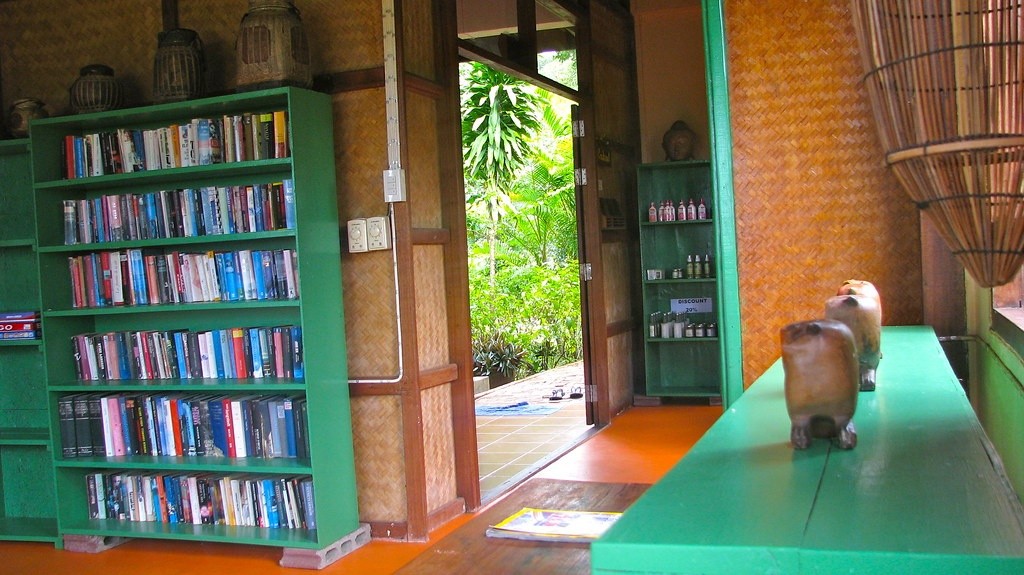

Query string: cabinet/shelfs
[634,159,722,398]
[0,87,359,549]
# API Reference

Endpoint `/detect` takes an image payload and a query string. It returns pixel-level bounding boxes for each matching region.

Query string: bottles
[650,310,690,338]
[695,255,702,278]
[705,254,710,278]
[686,255,694,278]
[649,201,657,222]
[664,200,670,221]
[670,200,675,221]
[678,200,686,221]
[698,198,706,220]
[688,198,696,220]
[658,201,664,222]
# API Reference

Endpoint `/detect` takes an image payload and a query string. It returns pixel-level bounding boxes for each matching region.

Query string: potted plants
[472,331,528,389]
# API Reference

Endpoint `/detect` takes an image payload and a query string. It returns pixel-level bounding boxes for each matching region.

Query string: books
[68,248,303,309]
[0,310,42,340]
[484,507,624,543]
[64,177,297,245]
[70,324,303,380]
[56,391,314,459]
[60,109,290,181]
[85,468,318,532]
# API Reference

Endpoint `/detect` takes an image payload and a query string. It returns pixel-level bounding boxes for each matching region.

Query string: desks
[592,324,1024,575]
